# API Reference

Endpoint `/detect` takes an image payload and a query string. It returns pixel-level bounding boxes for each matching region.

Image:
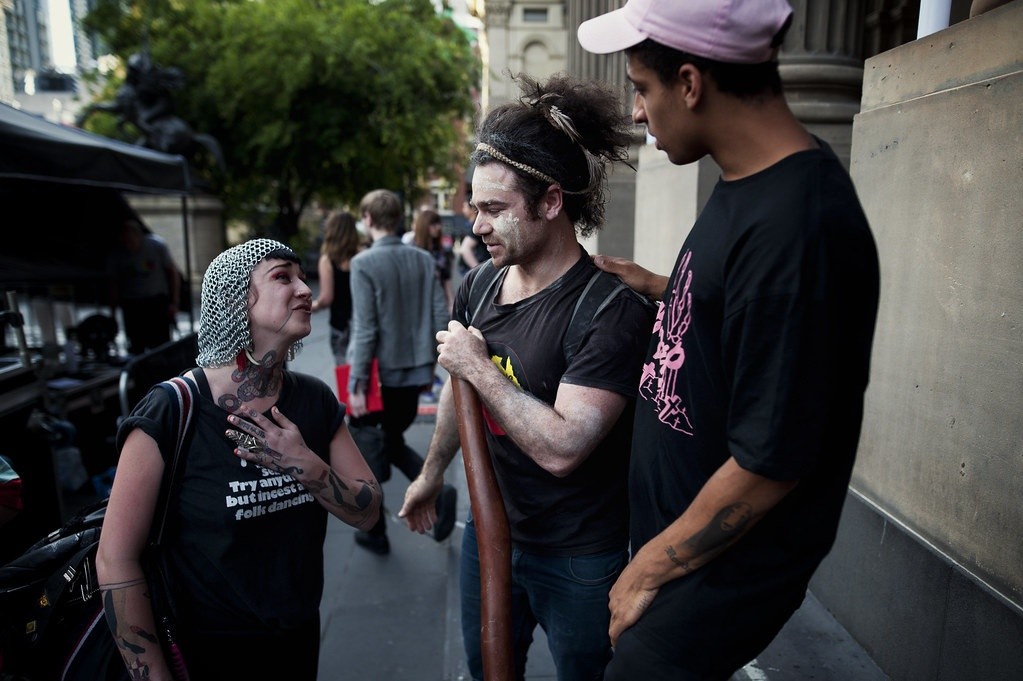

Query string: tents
[0,104,194,331]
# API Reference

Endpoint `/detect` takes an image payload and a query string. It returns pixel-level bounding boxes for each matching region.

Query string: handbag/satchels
[0,376,188,681]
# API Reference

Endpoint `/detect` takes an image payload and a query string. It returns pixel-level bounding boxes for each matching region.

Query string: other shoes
[428,485,457,542]
[352,530,391,555]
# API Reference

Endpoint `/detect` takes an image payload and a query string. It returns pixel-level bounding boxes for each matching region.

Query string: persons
[402,193,491,403]
[27,299,77,345]
[96,237,383,681]
[345,189,457,555]
[399,76,656,681]
[308,211,363,363]
[108,222,179,355]
[577,0,881,681]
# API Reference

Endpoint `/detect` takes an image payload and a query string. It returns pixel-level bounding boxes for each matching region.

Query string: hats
[576,0,793,62]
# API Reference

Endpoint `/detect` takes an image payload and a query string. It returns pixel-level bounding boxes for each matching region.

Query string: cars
[438,214,487,283]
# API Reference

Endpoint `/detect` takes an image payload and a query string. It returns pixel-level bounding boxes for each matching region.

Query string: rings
[235,431,263,450]
